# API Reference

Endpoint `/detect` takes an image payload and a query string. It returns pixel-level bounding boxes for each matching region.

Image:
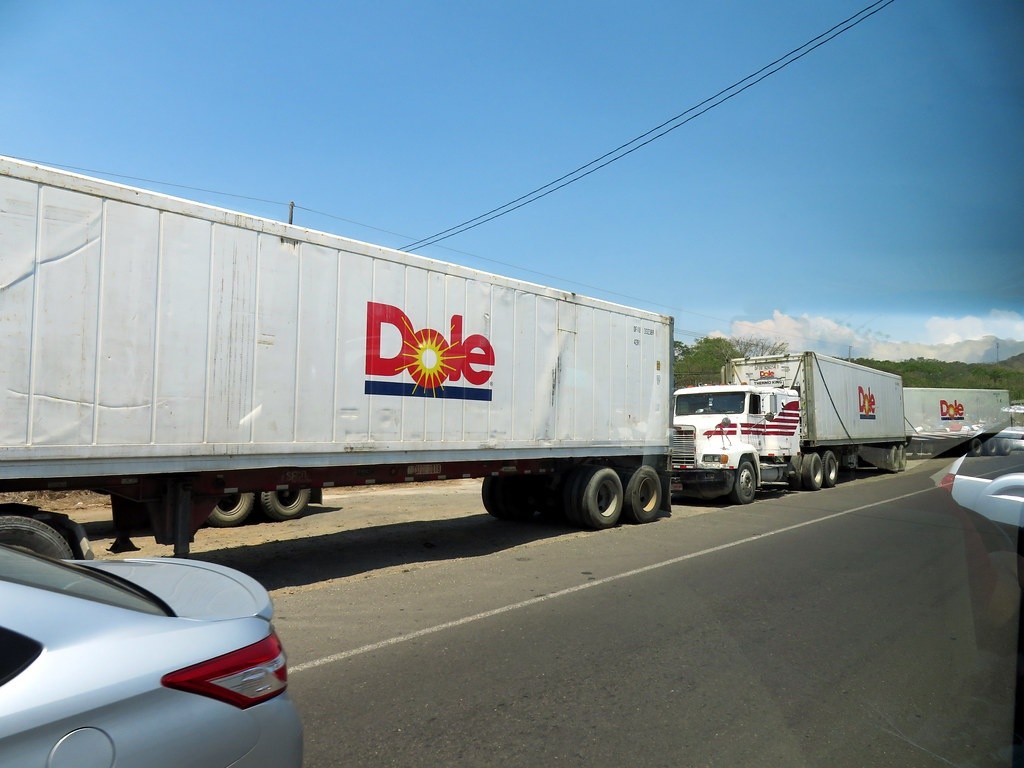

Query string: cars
[0,541,304,767]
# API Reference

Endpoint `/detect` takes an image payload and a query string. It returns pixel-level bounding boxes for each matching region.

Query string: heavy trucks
[667,350,906,505]
